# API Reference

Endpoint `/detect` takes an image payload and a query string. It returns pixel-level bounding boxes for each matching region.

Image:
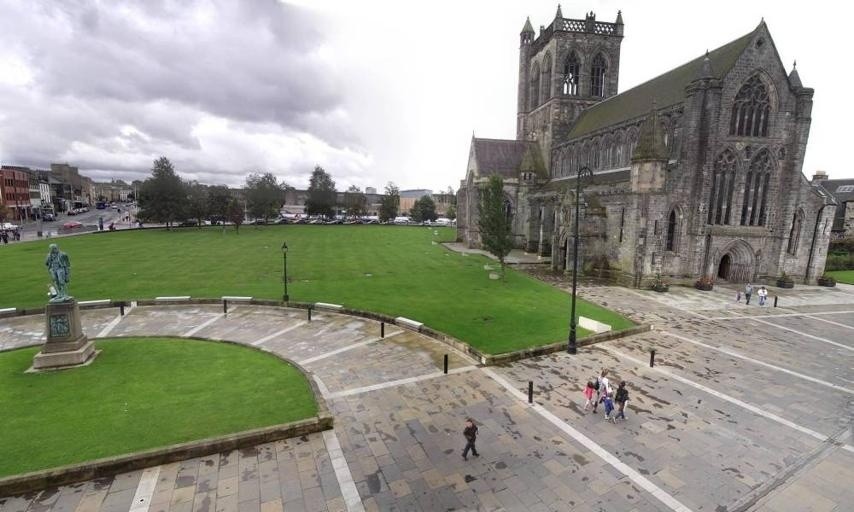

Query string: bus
[95,201,106,209]
[74,200,89,212]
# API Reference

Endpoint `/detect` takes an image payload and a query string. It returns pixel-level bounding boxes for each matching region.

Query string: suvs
[178,218,206,227]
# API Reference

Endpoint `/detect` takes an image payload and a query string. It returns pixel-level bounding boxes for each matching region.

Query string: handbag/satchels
[593,376,601,390]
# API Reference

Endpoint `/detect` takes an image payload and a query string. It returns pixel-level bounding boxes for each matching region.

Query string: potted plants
[776,269,796,289]
[649,270,672,294]
[694,276,716,291]
[816,275,836,288]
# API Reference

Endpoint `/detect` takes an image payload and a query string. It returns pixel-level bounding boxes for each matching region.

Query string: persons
[735,288,743,303]
[757,286,768,306]
[46,243,70,298]
[583,370,631,424]
[743,282,754,304]
[462,419,479,460]
[0,230,20,246]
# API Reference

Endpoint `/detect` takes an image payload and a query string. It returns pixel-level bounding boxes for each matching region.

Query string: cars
[250,218,396,224]
[111,200,133,209]
[68,208,80,215]
[57,224,84,234]
[44,213,55,221]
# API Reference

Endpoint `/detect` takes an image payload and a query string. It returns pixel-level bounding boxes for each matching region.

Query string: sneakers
[459,451,480,459]
[583,406,628,423]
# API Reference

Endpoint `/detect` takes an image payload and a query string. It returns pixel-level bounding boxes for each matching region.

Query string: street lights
[282,242,288,301]
[566,167,592,355]
